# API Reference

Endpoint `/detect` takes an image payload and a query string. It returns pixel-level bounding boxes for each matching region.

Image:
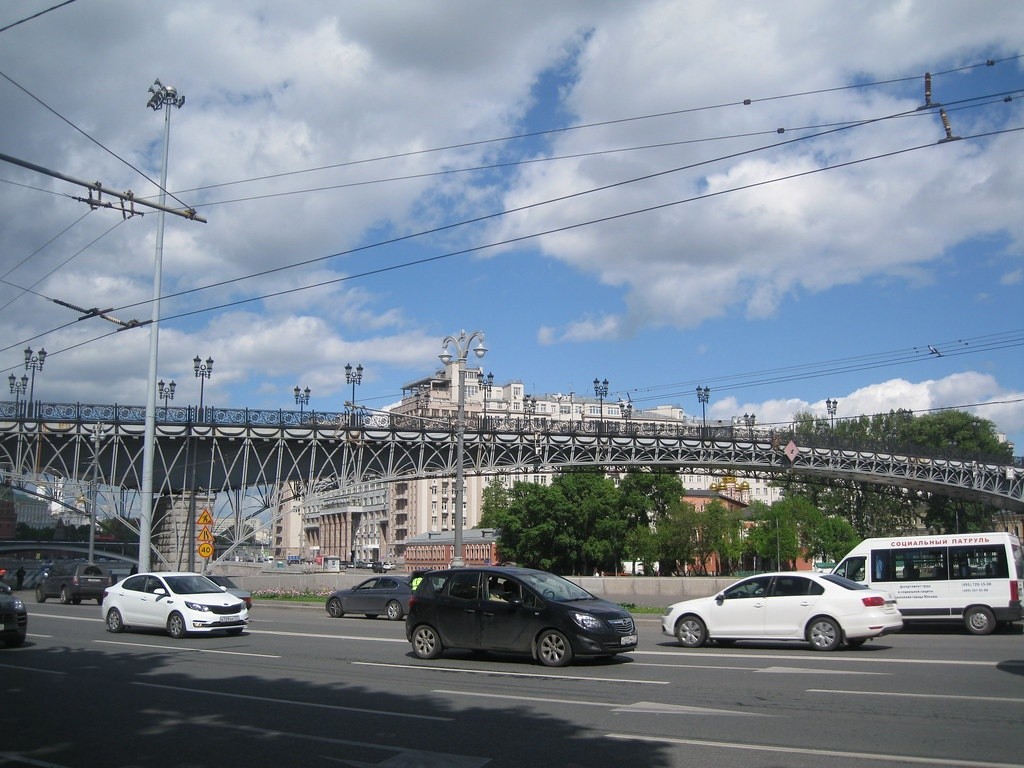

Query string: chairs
[502,580,518,600]
[881,559,998,580]
[487,580,500,600]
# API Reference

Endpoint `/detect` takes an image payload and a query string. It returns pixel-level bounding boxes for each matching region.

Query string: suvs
[36,563,108,605]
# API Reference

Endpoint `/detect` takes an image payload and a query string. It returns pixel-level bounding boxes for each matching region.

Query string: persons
[16,566,26,590]
[412,573,423,594]
[130,564,138,575]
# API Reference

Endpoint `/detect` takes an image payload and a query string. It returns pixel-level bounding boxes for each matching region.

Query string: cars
[660,571,903,652]
[341,560,395,573]
[102,572,252,638]
[326,576,411,621]
[0,578,27,648]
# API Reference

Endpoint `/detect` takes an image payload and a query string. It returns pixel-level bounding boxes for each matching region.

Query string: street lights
[345,363,363,423]
[438,330,494,569]
[294,385,311,425]
[137,78,185,573]
[695,385,756,451]
[523,397,537,432]
[593,378,631,444]
[971,419,981,469]
[8,346,47,417]
[158,355,214,422]
[825,398,838,467]
[903,408,912,465]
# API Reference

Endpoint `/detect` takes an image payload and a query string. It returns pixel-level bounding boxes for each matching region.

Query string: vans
[830,532,1024,635]
[405,567,638,666]
